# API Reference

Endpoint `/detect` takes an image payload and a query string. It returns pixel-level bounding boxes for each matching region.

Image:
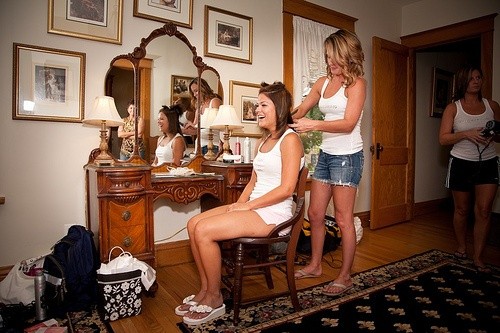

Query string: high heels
[174,294,199,315]
[183,302,226,325]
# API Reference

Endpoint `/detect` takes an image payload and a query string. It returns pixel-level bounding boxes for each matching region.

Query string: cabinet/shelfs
[83,162,255,273]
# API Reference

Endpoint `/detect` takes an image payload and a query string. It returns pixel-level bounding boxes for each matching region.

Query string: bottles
[235,138,241,162]
[244,136,251,163]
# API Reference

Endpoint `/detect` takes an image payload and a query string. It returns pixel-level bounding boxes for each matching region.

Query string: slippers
[294,269,320,279]
[321,281,352,296]
[454,248,468,259]
[472,263,492,275]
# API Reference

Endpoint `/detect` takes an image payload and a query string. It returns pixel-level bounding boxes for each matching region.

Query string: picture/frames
[12,42,86,123]
[170,75,197,107]
[229,80,266,138]
[133,0,194,30]
[47,0,125,45]
[430,67,457,118]
[204,5,253,64]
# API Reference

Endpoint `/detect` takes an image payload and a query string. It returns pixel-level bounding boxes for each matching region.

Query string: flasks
[35,270,47,321]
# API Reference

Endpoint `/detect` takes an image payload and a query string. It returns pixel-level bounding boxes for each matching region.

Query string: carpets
[176,247,500,333]
[0,271,115,333]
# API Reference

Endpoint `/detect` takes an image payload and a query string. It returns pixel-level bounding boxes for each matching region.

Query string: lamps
[202,108,220,158]
[83,96,123,167]
[211,105,245,162]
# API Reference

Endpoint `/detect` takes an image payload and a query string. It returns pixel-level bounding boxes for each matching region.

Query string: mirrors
[103,22,225,170]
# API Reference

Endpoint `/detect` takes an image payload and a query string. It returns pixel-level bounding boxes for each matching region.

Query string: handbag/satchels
[0,224,145,322]
[296,214,363,255]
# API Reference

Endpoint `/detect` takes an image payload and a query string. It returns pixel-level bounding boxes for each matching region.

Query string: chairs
[220,167,310,323]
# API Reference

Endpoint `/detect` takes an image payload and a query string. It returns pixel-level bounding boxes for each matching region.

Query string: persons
[439,63,500,274]
[150,104,187,168]
[288,28,368,296]
[179,77,223,156]
[175,81,306,325]
[117,100,144,160]
